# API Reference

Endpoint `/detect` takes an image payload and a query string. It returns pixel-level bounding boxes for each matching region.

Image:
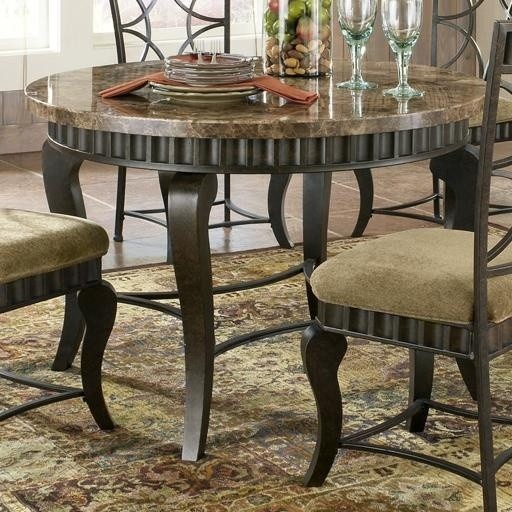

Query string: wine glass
[381,0,424,97]
[336,0,380,90]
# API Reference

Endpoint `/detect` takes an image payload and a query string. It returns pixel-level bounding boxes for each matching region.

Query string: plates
[149,53,267,103]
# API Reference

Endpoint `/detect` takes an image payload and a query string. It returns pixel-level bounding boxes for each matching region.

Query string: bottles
[264,0,332,78]
